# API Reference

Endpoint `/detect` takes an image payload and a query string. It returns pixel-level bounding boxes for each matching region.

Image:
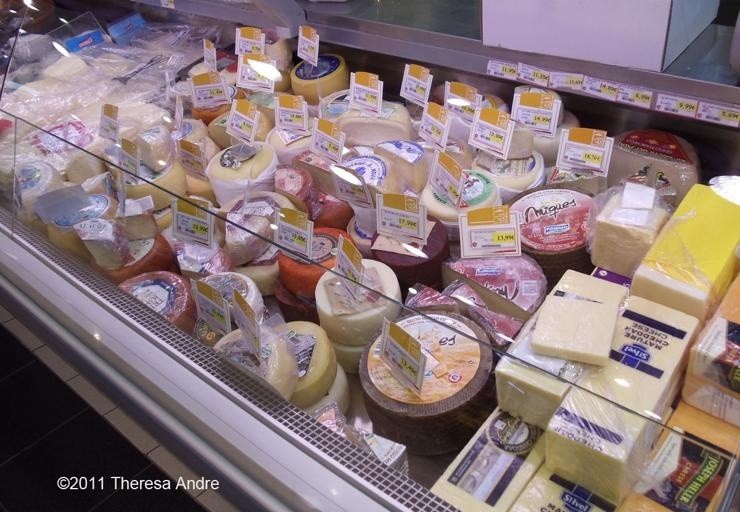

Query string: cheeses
[0,16,739,511]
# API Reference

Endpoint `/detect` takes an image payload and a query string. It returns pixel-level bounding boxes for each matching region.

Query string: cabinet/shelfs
[0,0,738,511]
